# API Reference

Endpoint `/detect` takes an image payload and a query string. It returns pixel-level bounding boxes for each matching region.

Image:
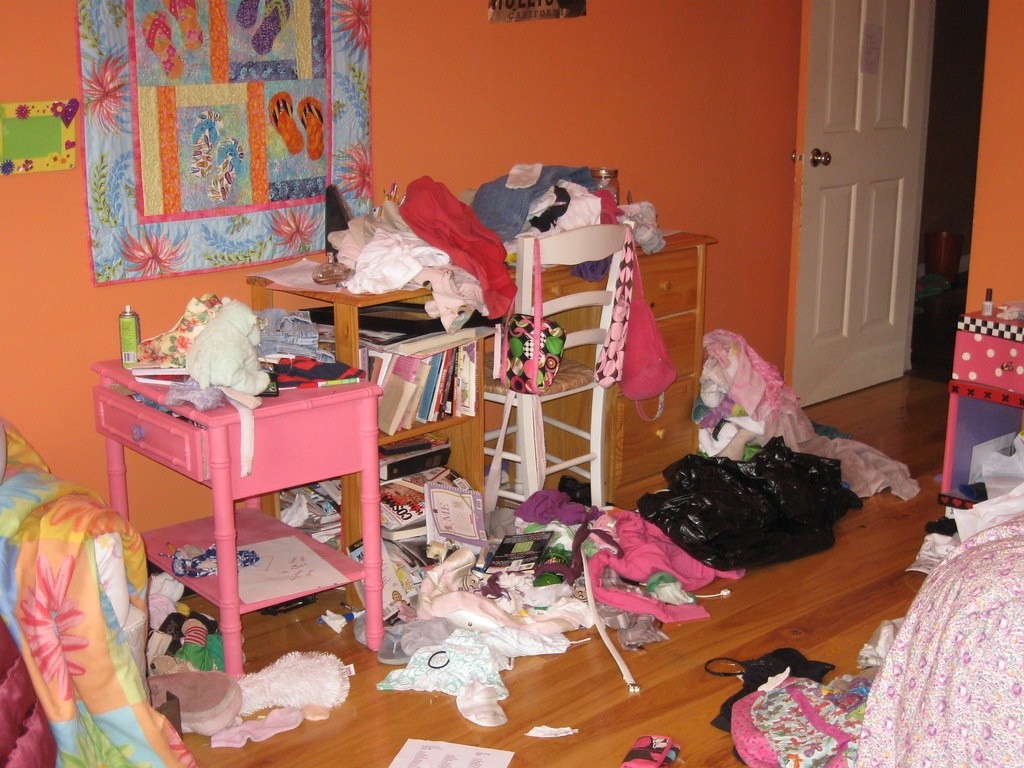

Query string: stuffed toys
[146,614,245,672]
[144,570,187,626]
[184,298,271,395]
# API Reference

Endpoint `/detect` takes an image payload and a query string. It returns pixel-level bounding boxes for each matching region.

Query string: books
[131,358,279,395]
[360,322,477,437]
[484,529,555,575]
[279,449,488,621]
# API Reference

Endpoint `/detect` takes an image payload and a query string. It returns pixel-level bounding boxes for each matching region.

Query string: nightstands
[937,379,1024,509]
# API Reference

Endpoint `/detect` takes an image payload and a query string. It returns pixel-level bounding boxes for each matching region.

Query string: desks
[90,360,383,679]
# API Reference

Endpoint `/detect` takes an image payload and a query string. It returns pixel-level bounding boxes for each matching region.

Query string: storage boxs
[952,306,1024,396]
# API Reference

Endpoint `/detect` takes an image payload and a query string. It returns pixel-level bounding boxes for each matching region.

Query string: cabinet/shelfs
[243,224,717,612]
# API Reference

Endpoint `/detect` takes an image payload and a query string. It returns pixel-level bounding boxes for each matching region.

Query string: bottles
[120,306,141,370]
[590,167,620,206]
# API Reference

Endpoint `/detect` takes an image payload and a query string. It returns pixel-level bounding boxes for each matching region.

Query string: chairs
[484,219,633,510]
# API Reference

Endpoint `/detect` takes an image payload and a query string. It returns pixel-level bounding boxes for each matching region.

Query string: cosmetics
[314,252,350,285]
